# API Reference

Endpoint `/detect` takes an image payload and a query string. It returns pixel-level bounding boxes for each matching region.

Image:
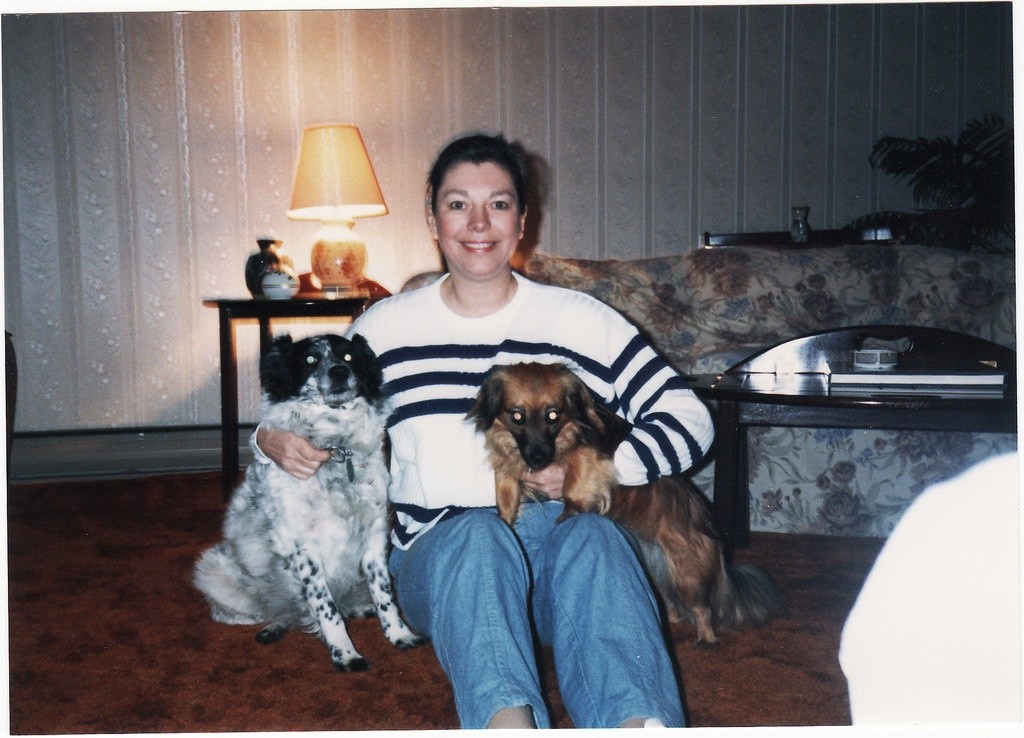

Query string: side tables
[203,298,380,502]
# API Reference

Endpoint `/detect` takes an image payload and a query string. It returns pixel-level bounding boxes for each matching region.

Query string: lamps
[288,126,388,294]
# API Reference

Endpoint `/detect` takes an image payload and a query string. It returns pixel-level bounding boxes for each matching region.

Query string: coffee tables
[684,330,1017,571]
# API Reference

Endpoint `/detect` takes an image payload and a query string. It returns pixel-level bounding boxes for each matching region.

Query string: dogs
[191,332,431,674]
[465,360,782,649]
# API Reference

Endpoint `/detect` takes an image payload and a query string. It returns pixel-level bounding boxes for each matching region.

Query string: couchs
[401,242,1017,538]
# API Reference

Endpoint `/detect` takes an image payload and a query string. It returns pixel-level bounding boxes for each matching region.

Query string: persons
[250,133,714,730]
